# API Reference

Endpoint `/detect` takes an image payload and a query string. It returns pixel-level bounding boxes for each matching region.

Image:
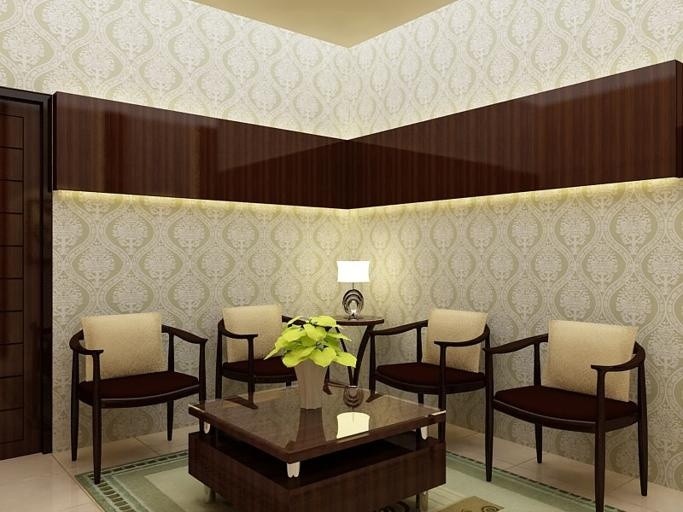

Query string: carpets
[74,452,629,512]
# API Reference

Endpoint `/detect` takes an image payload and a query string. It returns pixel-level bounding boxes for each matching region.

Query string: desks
[331,314,384,386]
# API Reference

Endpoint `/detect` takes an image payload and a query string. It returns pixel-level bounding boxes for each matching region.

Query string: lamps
[336,259,370,320]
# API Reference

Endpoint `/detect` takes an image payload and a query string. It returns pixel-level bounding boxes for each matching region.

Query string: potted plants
[269,318,358,409]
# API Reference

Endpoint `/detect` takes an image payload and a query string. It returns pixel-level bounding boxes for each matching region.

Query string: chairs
[368,318,495,483]
[69,324,207,485]
[215,315,333,400]
[482,332,648,512]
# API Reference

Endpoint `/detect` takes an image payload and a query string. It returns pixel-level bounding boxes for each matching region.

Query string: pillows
[540,319,640,403]
[420,308,489,373]
[223,303,283,364]
[81,309,167,382]
